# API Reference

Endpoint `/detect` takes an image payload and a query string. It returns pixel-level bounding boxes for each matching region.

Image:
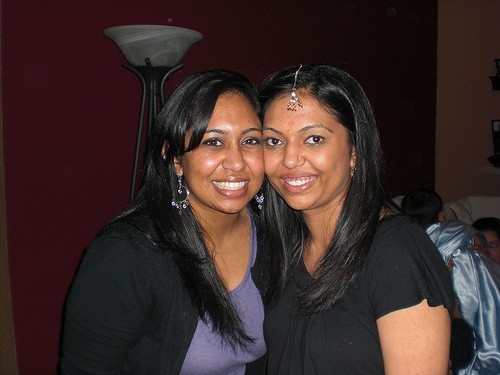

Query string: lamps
[102,24,203,207]
[489,58,500,91]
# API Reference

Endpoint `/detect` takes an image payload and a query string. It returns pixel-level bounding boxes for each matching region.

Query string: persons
[400,189,500,375]
[467,217,500,265]
[257,64,455,374]
[57,69,267,375]
